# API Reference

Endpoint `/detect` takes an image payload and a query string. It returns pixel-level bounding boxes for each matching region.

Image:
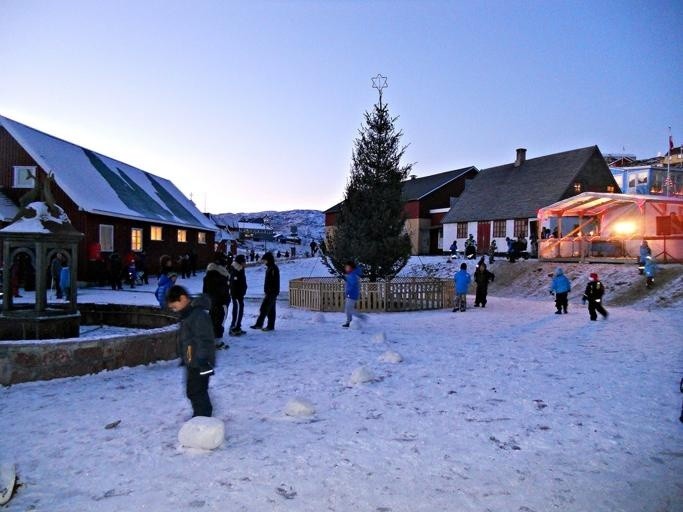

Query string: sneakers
[230,329,241,336]
[554,311,561,314]
[215,342,230,350]
[342,323,349,327]
[237,328,247,335]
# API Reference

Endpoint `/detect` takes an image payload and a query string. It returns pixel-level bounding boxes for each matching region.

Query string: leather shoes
[250,326,261,329]
[262,328,270,331]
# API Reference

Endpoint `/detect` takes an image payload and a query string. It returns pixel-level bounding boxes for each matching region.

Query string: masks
[588,277,594,282]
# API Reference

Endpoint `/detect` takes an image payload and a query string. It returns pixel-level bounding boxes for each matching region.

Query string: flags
[669,131,674,152]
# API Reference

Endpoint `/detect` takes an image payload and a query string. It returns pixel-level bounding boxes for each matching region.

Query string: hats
[167,272,177,278]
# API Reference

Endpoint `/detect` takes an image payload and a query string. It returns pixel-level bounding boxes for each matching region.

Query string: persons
[229,254,248,337]
[249,251,280,332]
[549,266,571,314]
[0,235,197,302]
[341,261,369,327]
[449,241,457,262]
[202,250,231,351]
[581,272,609,321]
[473,262,495,307]
[465,234,476,260]
[505,226,562,263]
[644,255,655,289]
[165,285,216,417]
[452,263,471,312]
[638,241,651,275]
[244,236,320,261]
[488,240,497,264]
